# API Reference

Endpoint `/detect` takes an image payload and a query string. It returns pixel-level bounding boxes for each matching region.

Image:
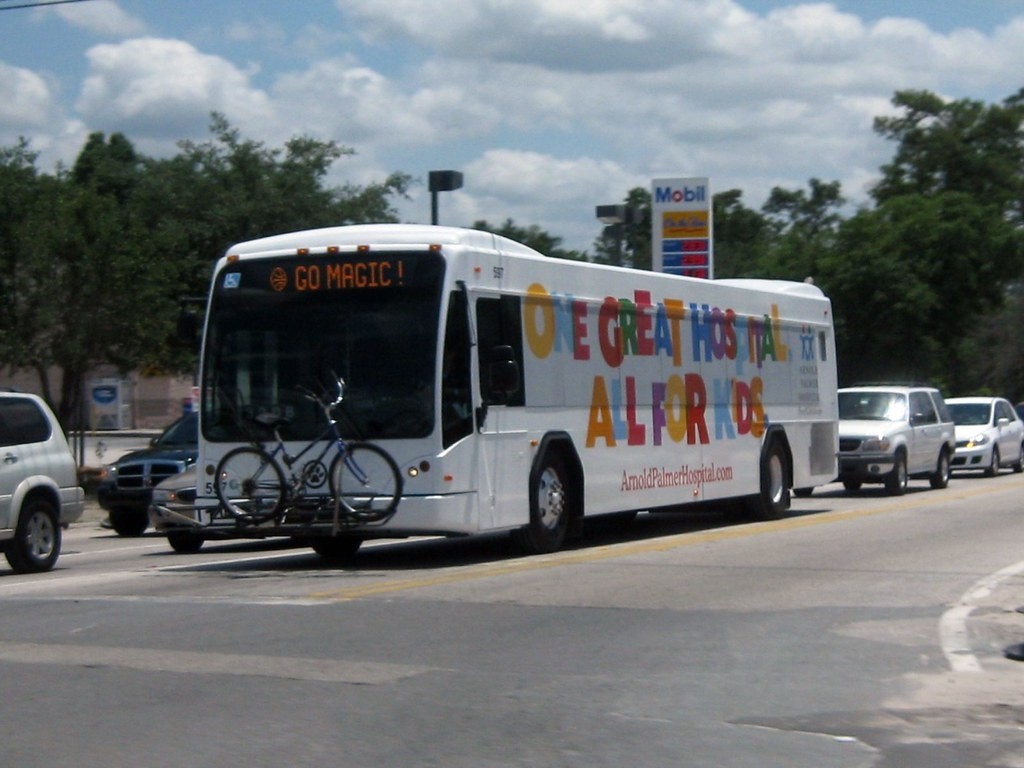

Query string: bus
[193,224,840,553]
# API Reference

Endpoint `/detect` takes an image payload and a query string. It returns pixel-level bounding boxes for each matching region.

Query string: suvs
[98,411,200,536]
[0,385,84,574]
[795,383,955,498]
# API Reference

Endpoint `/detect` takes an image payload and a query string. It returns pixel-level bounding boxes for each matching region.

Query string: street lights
[429,169,464,225]
[596,204,633,270]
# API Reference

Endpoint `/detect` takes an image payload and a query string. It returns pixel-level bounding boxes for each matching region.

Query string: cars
[942,397,1024,478]
[153,457,310,552]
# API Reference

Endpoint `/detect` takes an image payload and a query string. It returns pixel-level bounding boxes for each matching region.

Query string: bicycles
[216,370,405,522]
